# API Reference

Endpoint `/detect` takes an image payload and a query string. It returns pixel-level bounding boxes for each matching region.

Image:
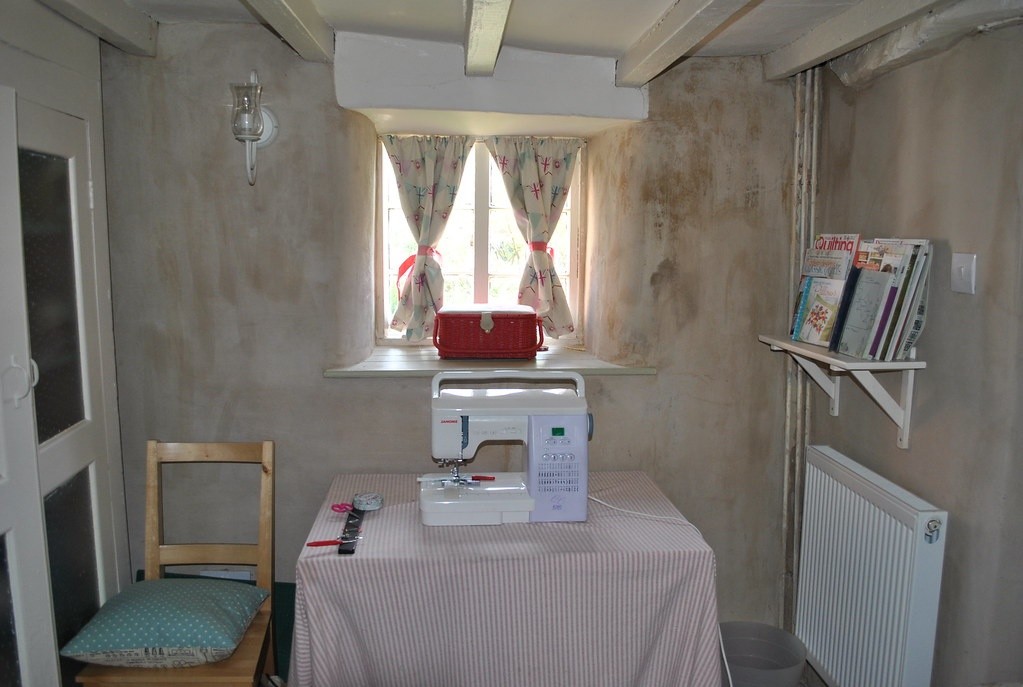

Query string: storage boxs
[433,304,544,359]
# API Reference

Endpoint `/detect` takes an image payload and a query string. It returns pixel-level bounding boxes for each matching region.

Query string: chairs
[59,439,277,687]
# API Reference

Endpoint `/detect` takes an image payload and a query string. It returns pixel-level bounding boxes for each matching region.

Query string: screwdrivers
[306,540,357,547]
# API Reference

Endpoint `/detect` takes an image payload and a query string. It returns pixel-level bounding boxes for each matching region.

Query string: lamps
[228,71,279,187]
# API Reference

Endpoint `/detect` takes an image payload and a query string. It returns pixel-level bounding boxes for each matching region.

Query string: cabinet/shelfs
[0,84,134,687]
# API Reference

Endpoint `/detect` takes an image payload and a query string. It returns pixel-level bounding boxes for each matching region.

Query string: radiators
[794,445,949,687]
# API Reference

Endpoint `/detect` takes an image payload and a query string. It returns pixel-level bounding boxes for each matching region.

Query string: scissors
[330,503,360,519]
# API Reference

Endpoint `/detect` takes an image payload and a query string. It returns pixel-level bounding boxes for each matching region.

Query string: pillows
[59,579,271,669]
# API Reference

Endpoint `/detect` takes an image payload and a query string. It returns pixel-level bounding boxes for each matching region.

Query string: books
[791,234,933,361]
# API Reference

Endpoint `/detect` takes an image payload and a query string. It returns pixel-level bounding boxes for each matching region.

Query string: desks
[287,470,721,687]
